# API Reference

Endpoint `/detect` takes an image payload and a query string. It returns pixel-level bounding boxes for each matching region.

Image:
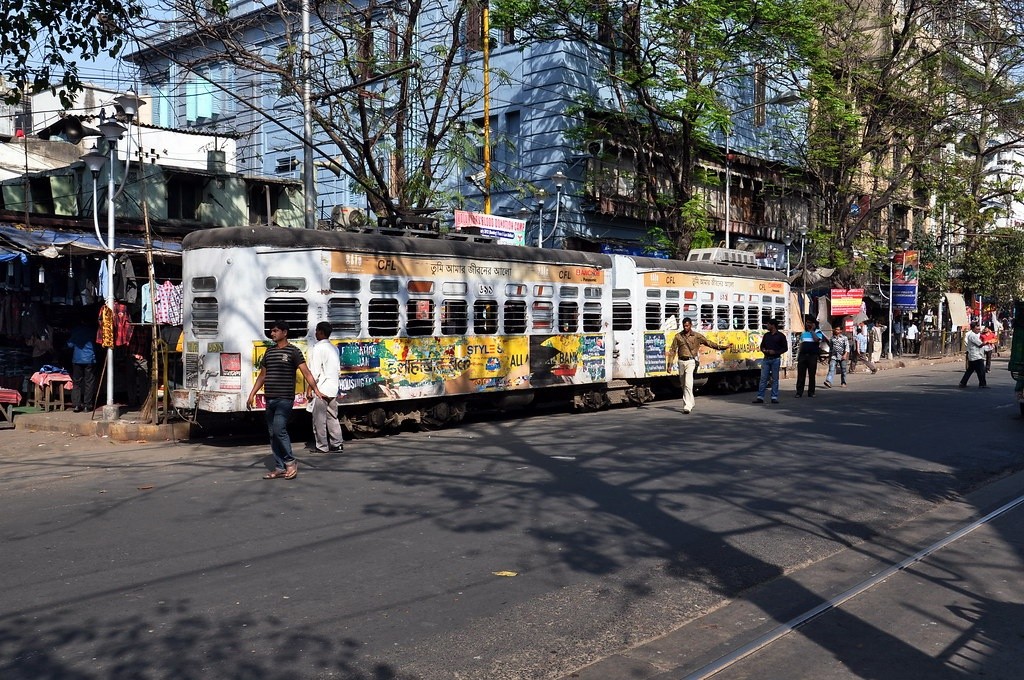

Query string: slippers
[285,460,298,479]
[263,470,285,479]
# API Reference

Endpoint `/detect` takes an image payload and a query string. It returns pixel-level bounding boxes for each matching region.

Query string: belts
[678,356,694,361]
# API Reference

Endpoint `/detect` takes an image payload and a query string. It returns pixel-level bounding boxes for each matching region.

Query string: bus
[167,59,794,444]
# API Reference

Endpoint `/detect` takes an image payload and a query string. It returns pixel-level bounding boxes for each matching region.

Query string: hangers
[91,250,183,311]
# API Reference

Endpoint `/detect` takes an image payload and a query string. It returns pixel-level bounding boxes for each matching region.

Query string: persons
[125,327,149,407]
[849,330,877,374]
[246,320,331,479]
[893,319,901,356]
[980,326,997,373]
[859,318,874,361]
[66,324,98,412]
[667,317,733,412]
[795,317,822,398]
[717,316,726,329]
[26,325,54,372]
[871,320,887,363]
[703,316,712,329]
[959,325,995,389]
[1002,316,1013,337]
[964,322,974,370]
[824,326,850,388]
[752,319,788,403]
[904,320,918,354]
[306,322,344,453]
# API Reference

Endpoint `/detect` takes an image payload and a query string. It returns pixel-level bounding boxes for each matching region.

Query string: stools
[29,371,73,413]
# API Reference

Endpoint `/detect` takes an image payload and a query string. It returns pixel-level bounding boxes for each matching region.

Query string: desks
[0,388,23,429]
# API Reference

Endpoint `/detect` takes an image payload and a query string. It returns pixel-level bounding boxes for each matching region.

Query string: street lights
[875,239,912,353]
[79,83,149,406]
[725,87,803,249]
[768,222,811,277]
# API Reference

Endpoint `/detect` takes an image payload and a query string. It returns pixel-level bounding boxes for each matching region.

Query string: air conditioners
[585,139,604,158]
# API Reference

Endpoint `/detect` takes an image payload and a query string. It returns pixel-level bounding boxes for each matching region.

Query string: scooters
[1008,367,1024,419]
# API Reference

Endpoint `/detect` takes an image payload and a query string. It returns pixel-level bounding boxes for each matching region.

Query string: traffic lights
[14,111,24,137]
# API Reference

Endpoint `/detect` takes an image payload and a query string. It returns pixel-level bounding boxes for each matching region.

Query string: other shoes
[771,399,779,403]
[795,394,802,398]
[330,445,344,451]
[752,399,763,403]
[683,409,689,414]
[847,371,854,374]
[311,448,330,454]
[808,393,816,398]
[871,369,878,374]
[824,381,831,388]
[83,407,92,412]
[73,405,82,412]
[959,385,968,390]
[979,385,991,389]
[841,383,847,388]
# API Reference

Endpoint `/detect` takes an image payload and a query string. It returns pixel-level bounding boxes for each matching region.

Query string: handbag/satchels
[694,361,699,374]
